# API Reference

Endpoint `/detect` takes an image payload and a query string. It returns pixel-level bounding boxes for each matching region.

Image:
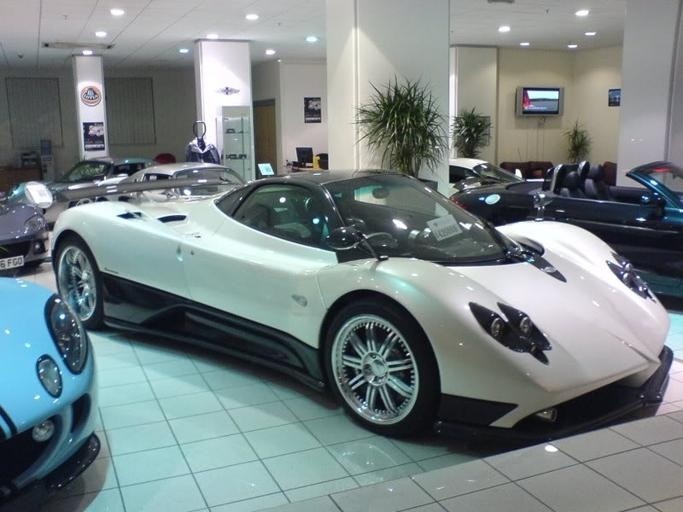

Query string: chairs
[246,197,330,241]
[560,163,612,200]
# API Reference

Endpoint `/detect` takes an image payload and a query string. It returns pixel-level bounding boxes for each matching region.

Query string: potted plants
[350,72,450,215]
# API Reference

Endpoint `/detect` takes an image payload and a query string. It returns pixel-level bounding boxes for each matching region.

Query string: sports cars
[55,170,674,441]
[449,161,682,313]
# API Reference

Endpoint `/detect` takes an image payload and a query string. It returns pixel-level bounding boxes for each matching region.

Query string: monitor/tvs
[516,85,563,118]
[296,146,313,162]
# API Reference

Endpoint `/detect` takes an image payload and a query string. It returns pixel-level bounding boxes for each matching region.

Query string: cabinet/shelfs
[0,166,42,191]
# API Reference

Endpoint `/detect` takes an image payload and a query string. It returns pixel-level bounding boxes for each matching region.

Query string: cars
[448,157,523,196]
[0,276,102,507]
[0,153,246,279]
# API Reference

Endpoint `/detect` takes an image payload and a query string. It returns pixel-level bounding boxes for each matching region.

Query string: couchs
[500,161,554,178]
